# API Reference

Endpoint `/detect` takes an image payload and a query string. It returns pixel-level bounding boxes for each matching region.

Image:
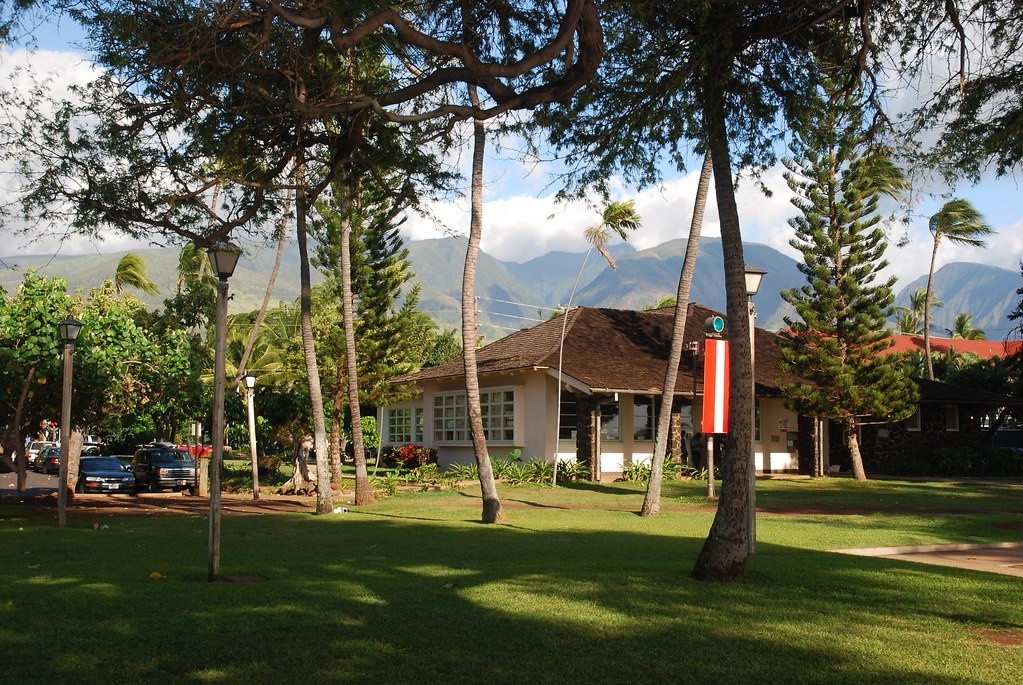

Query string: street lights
[56,314,85,527]
[205,234,243,582]
[239,371,261,501]
[744,261,769,556]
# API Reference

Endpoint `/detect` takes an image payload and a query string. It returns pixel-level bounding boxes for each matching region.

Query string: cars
[34,445,62,474]
[74,456,137,495]
[25,434,106,468]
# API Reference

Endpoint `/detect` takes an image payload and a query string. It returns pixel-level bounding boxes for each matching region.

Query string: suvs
[126,440,201,496]
